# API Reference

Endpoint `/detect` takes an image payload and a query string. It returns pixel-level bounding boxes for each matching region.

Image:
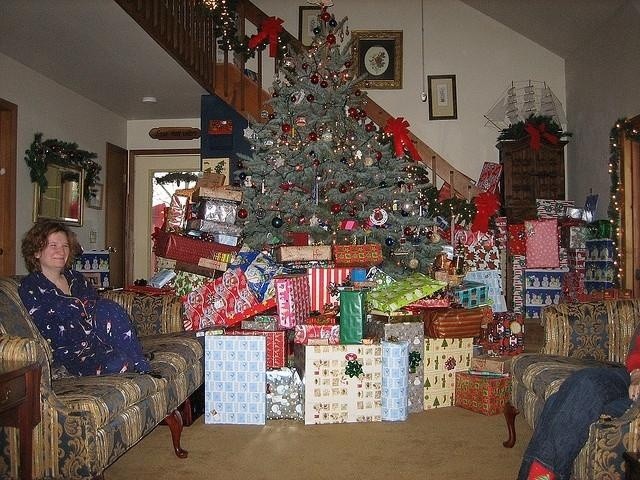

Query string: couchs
[0,272,205,480]
[505,296,640,480]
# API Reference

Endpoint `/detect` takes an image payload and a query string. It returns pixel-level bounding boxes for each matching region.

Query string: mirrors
[31,161,86,227]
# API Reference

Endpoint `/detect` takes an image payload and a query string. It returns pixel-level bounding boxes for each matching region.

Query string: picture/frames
[428,74,457,120]
[351,29,403,91]
[86,182,104,210]
[298,6,323,50]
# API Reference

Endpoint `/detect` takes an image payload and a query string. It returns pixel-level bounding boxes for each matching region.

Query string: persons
[517,323,640,479]
[18,220,153,376]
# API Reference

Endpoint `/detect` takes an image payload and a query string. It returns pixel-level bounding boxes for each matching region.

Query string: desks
[0,359,41,480]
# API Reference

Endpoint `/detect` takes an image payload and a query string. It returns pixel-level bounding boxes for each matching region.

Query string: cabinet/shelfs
[496,140,570,211]
